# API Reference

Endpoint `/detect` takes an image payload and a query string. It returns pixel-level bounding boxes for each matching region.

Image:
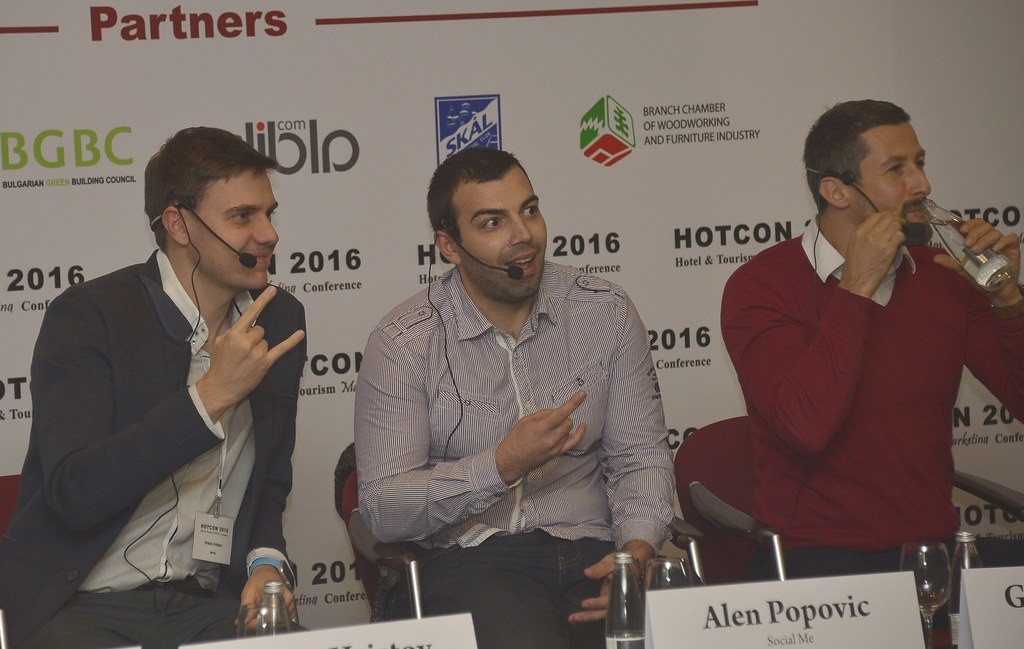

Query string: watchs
[250,558,295,591]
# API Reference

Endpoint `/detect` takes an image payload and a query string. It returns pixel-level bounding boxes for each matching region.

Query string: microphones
[184,202,257,267]
[848,182,926,236]
[452,236,524,280]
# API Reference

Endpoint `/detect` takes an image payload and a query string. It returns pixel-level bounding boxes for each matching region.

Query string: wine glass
[900,541,953,649]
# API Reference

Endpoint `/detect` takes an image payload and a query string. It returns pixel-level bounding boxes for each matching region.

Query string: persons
[720,99,1024,631]
[0,127,306,649]
[354,147,674,649]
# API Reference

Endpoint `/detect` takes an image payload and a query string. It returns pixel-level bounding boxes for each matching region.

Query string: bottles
[949,531,983,649]
[920,199,1014,292]
[258,580,291,638]
[606,552,646,649]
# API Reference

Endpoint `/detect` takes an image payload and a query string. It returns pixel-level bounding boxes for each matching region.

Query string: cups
[237,603,281,639]
[643,557,693,600]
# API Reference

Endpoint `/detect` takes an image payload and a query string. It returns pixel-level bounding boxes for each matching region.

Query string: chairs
[334,438,706,619]
[673,416,1024,580]
[0,473,297,649]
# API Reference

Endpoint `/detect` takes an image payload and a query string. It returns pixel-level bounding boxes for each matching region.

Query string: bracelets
[987,284,1024,320]
[633,558,645,585]
